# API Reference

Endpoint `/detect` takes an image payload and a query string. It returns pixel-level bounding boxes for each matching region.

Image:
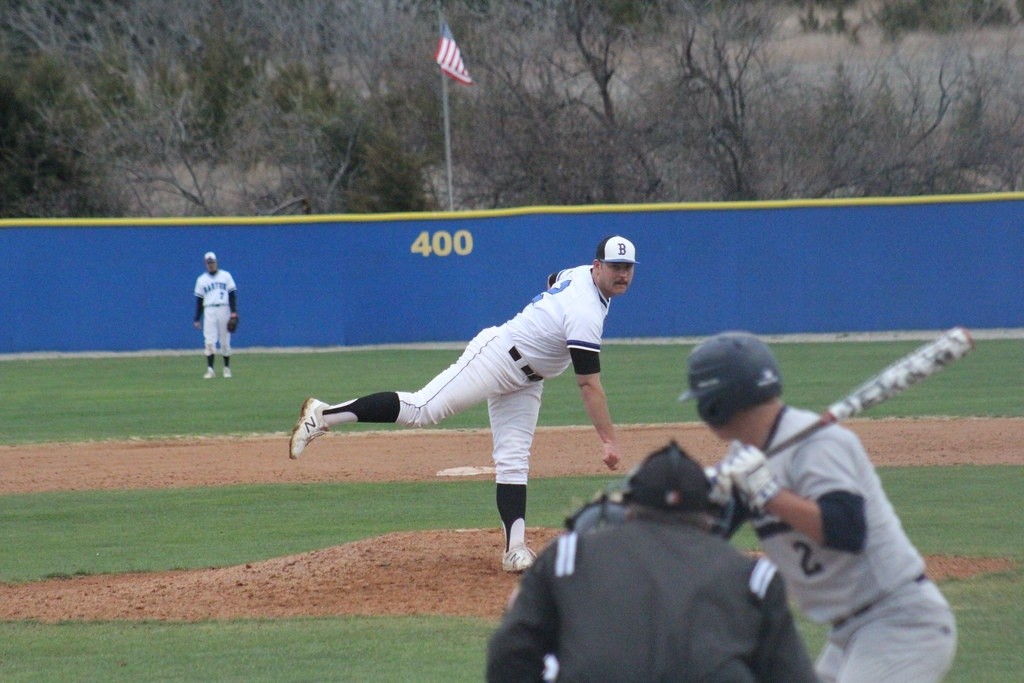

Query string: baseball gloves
[228,317,238,332]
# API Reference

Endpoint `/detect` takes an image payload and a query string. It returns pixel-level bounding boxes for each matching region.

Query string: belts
[508,346,543,383]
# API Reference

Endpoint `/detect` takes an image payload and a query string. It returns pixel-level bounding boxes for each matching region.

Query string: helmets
[678,332,786,428]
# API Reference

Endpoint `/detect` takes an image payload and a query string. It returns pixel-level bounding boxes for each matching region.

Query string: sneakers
[502,546,534,573]
[289,397,329,460]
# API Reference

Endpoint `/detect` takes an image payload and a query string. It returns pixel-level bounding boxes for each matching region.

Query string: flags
[434,21,472,85]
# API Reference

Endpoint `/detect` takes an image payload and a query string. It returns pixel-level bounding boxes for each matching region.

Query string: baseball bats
[766,323,975,459]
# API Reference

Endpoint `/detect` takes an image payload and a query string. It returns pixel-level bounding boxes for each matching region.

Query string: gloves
[719,440,781,507]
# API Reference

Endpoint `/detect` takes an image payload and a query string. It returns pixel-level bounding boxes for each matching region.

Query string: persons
[486,444,824,683]
[568,331,957,683]
[290,234,639,572]
[194,252,237,378]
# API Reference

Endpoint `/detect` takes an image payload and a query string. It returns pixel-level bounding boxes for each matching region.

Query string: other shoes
[223,366,231,378]
[204,367,215,378]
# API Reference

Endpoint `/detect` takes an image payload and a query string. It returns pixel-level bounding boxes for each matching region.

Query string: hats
[204,251,216,260]
[596,234,640,264]
[625,440,712,511]
